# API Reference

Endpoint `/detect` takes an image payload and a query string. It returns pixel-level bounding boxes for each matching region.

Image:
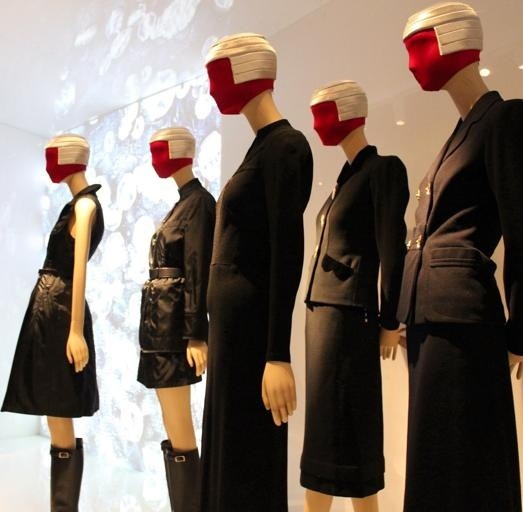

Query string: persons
[298,77,410,512]
[131,122,218,512]
[391,2,522,511]
[0,132,106,510]
[187,29,312,510]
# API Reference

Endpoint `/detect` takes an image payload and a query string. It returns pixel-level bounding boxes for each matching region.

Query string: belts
[149,267,183,280]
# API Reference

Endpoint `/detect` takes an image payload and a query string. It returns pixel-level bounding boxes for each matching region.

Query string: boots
[161,440,200,512]
[50,437,83,512]
